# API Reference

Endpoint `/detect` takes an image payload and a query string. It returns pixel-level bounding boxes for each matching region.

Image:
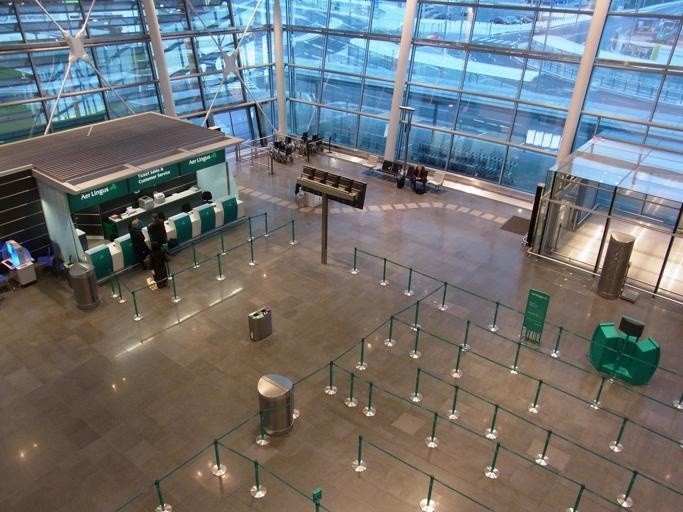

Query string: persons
[411,163,427,192]
[278,140,286,150]
[129,213,170,289]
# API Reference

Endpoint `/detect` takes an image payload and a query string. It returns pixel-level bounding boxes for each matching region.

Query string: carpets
[500,215,531,237]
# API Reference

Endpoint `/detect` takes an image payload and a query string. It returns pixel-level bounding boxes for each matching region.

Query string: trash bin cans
[257,373,295,436]
[63,262,102,311]
[247,305,273,341]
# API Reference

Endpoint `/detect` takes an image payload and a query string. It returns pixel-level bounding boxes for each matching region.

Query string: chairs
[362,155,446,193]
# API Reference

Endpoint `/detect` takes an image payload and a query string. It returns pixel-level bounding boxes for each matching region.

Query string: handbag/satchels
[146,277,158,291]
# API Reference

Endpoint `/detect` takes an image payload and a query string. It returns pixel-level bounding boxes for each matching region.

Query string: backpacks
[166,237,181,257]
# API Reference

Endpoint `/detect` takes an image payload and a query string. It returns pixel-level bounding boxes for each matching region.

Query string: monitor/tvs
[619,315,645,337]
